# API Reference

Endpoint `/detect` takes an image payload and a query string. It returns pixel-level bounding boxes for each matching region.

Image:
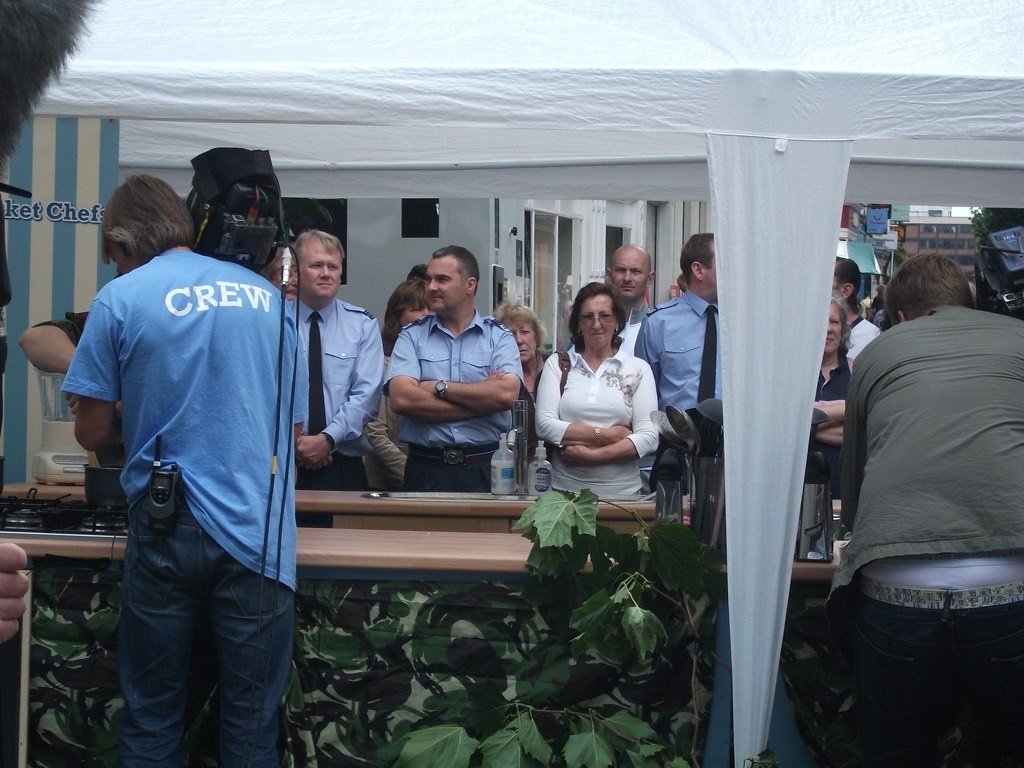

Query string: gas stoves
[0,496,130,544]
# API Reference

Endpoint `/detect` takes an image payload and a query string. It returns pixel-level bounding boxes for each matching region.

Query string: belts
[408,449,497,469]
[859,572,1024,608]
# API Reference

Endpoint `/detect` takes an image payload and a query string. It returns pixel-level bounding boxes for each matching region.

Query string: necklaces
[813,290,856,500]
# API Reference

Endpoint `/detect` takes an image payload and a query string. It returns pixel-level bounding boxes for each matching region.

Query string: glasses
[576,313,619,326]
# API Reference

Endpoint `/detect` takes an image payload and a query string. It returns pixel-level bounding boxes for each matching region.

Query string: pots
[82,462,129,508]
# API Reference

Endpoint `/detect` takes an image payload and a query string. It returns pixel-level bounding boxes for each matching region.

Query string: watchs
[435,379,448,400]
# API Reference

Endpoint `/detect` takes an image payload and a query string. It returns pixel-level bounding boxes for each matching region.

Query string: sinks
[360,490,525,503]
[521,494,654,504]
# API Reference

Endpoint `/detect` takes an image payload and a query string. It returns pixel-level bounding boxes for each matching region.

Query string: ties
[309,311,326,437]
[698,304,719,404]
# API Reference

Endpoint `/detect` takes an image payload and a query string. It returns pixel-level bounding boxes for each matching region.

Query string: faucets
[507,399,530,495]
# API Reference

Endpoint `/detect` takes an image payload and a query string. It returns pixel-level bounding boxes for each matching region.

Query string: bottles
[490,434,516,494]
[528,440,553,495]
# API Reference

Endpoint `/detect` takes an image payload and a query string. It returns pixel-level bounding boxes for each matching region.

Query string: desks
[0,477,691,768]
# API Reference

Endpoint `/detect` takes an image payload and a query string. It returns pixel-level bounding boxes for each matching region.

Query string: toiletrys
[527,440,553,494]
[490,433,516,495]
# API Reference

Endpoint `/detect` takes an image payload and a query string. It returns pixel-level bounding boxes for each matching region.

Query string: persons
[535,282,659,496]
[60,173,309,768]
[18,311,126,467]
[634,233,723,496]
[832,255,883,360]
[871,284,886,312]
[0,542,29,644]
[261,241,297,301]
[361,278,437,492]
[492,300,552,465]
[607,244,656,356]
[857,291,873,319]
[287,228,384,528]
[383,245,525,493]
[825,253,1024,768]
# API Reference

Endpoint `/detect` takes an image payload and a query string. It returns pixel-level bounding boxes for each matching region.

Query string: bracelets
[322,432,334,450]
[594,427,600,445]
